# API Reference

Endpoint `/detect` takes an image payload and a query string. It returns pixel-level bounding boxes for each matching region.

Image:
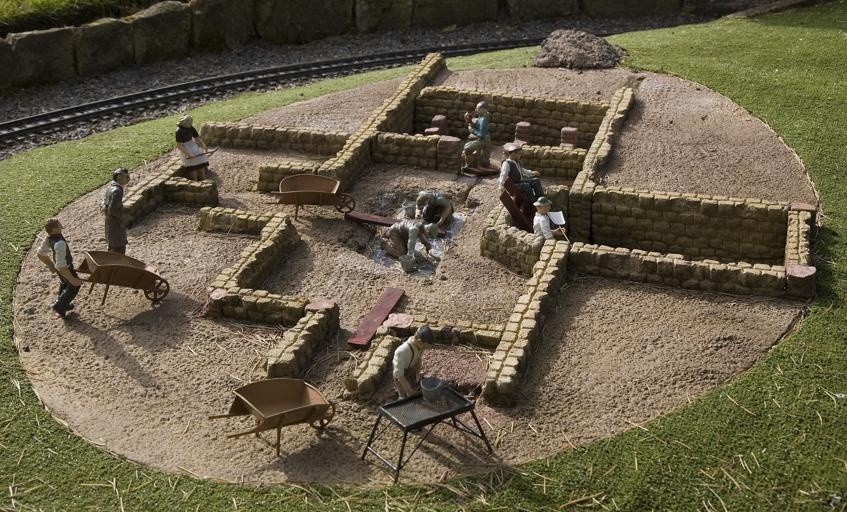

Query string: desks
[362,385,492,484]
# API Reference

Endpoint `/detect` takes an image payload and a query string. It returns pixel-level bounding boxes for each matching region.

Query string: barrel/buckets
[399,253,416,274]
[402,199,417,218]
[422,374,443,402]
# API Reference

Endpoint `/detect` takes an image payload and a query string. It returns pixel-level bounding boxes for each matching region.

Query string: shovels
[546,211,573,243]
[187,147,226,157]
